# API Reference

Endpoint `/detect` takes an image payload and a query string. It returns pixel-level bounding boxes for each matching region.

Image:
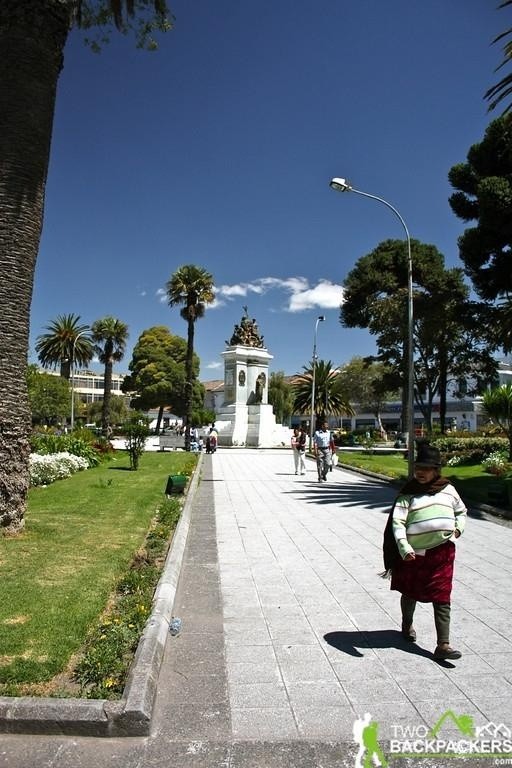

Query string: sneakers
[299,450,304,454]
[402,623,416,642]
[435,646,462,660]
[319,473,327,482]
[295,471,305,475]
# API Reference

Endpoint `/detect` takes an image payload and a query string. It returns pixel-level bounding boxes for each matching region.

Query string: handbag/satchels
[331,453,338,465]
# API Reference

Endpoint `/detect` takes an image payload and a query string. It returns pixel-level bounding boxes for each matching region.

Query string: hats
[412,445,442,467]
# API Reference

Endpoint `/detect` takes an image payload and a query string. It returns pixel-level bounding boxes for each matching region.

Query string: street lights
[61,328,97,433]
[329,173,416,480]
[307,313,327,455]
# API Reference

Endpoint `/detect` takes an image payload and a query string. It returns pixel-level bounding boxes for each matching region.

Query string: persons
[169,417,218,453]
[313,420,333,482]
[291,427,307,475]
[390,442,467,662]
[297,428,307,454]
[327,429,337,471]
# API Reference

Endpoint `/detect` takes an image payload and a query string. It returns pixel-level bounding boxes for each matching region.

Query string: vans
[83,423,95,429]
